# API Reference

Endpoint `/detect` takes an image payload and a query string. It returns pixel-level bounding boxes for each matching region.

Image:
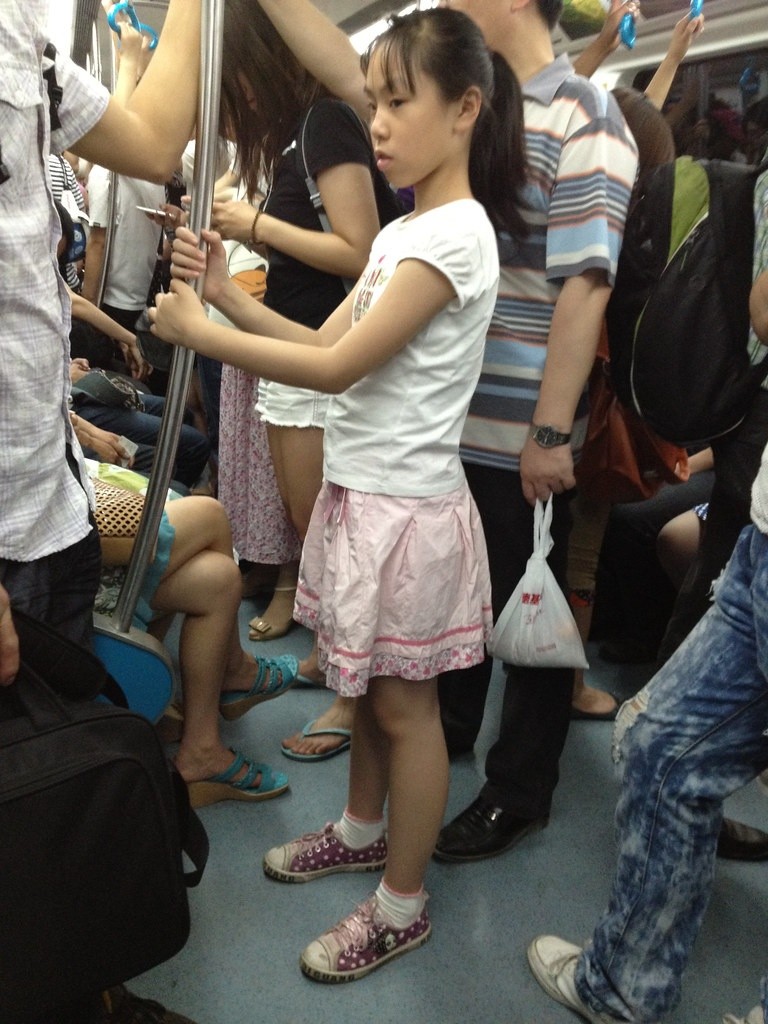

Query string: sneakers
[527,934,613,1024]
[298,891,432,983]
[264,821,387,883]
[723,1006,765,1024]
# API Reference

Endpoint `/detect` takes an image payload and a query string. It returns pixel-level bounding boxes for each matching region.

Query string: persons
[0,0,414,1024]
[430,1,768,860]
[147,6,530,983]
[527,270,768,1024]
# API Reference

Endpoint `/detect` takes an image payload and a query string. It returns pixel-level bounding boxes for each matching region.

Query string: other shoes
[83,982,196,1024]
[241,571,271,597]
[570,688,632,718]
[247,586,301,641]
[715,816,768,860]
[600,639,636,669]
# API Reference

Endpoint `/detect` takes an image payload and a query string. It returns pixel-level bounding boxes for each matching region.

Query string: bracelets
[251,210,264,245]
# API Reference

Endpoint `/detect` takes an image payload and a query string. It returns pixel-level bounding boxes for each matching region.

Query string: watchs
[529,422,572,447]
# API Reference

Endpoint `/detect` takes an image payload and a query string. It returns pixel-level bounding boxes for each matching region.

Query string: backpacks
[615,154,768,449]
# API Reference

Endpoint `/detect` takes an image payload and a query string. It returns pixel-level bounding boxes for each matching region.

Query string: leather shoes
[443,739,474,760]
[431,793,550,861]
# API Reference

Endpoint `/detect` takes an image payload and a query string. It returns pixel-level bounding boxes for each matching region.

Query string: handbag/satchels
[2,608,209,1019]
[56,154,82,225]
[577,306,689,504]
[486,490,591,669]
[146,261,175,308]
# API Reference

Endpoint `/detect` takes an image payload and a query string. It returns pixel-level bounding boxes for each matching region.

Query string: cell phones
[135,206,176,220]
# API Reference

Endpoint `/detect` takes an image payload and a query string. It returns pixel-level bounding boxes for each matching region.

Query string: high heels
[184,744,288,809]
[220,654,299,722]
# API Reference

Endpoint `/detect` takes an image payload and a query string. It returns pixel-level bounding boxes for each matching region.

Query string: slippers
[295,659,327,688]
[282,719,353,761]
